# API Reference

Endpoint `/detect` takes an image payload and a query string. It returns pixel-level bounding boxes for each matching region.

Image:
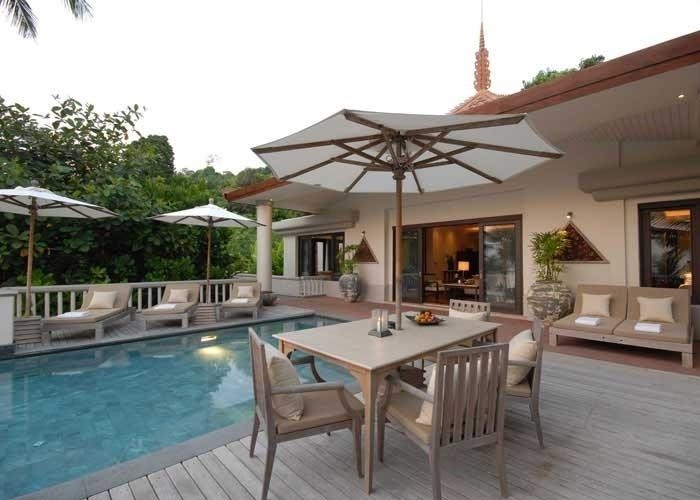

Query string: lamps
[457,261,470,283]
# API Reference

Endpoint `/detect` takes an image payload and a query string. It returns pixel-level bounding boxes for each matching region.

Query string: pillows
[88,290,118,311]
[167,287,190,303]
[415,357,501,428]
[506,328,537,386]
[236,285,255,298]
[262,338,306,423]
[450,309,487,347]
[637,294,675,323]
[579,292,615,318]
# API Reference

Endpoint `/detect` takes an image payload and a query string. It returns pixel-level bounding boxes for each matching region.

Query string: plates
[405,314,444,326]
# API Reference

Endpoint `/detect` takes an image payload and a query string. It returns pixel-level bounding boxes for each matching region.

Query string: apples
[416,311,436,323]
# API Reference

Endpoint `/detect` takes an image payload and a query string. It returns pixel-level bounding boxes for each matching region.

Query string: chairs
[214,280,265,321]
[139,282,202,332]
[40,283,139,342]
[496,317,551,449]
[419,300,492,369]
[403,268,506,303]
[380,342,510,499]
[244,324,365,499]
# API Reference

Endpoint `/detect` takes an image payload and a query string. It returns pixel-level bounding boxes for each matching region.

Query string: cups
[372,309,389,332]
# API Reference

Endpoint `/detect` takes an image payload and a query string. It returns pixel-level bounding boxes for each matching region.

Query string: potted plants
[526,230,573,327]
[335,244,368,303]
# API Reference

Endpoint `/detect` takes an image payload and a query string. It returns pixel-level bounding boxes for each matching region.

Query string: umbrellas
[252,109,563,394]
[0,182,119,316]
[146,198,267,302]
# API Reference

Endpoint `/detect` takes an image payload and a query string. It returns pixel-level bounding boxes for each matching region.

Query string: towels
[574,316,602,328]
[633,322,665,333]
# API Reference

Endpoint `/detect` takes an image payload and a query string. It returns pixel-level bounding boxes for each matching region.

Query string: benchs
[548,280,696,371]
